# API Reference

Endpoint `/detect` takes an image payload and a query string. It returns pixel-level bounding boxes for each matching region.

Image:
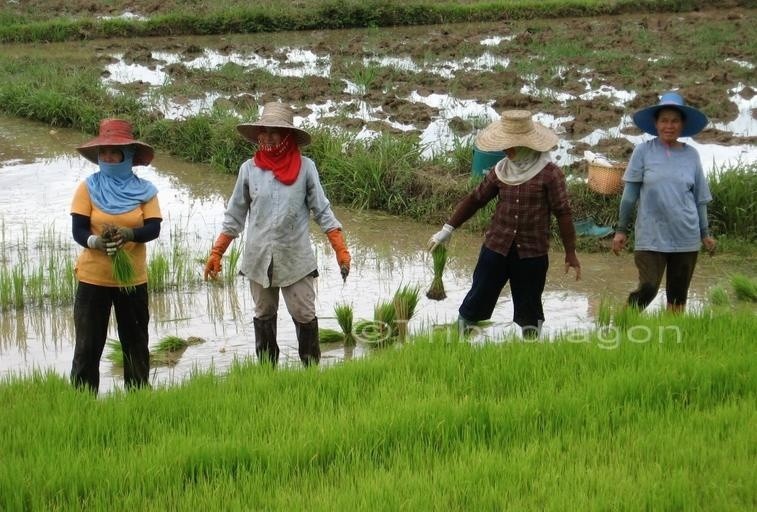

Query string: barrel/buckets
[471,150,507,179]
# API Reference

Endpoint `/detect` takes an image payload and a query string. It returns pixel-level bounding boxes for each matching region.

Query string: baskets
[587,154,628,195]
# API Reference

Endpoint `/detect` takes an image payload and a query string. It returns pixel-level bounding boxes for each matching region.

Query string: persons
[611,92,717,315]
[70,119,163,399]
[426,109,582,338]
[204,102,351,368]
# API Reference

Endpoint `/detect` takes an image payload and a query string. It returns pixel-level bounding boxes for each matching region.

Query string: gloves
[426,224,456,251]
[202,232,233,282]
[326,228,353,274]
[86,226,135,254]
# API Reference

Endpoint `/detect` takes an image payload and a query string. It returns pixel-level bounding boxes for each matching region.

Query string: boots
[291,315,323,370]
[254,313,281,369]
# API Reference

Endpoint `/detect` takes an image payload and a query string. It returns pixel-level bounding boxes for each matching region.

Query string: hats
[75,117,155,170]
[630,91,710,138]
[237,101,313,147]
[474,107,559,153]
